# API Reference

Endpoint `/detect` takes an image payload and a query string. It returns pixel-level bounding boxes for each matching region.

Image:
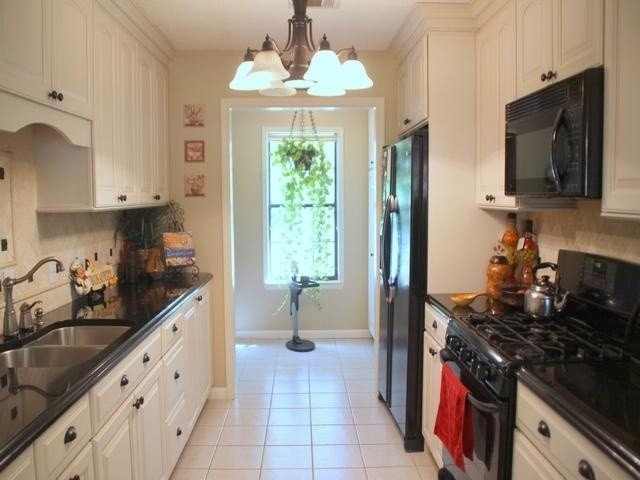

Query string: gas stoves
[459,313,631,362]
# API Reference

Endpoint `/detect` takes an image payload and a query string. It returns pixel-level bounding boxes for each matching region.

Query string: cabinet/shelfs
[509,370,640,479]
[159,308,186,480]
[397,33,429,137]
[183,280,212,427]
[1,1,93,148]
[92,0,135,211]
[135,42,170,208]
[601,1,640,221]
[516,0,603,100]
[419,296,448,470]
[477,0,515,195]
[90,328,162,478]
[1,394,94,480]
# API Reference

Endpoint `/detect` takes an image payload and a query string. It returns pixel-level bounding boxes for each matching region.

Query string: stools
[285,274,320,351]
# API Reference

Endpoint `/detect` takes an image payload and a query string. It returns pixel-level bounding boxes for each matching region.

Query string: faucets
[8,367,71,400]
[2,256,65,337]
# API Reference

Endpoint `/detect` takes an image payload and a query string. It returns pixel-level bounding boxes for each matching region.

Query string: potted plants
[268,107,333,309]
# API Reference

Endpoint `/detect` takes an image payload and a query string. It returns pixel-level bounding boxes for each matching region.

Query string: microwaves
[503,66,603,199]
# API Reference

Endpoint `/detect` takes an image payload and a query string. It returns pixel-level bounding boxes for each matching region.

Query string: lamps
[229,0,374,97]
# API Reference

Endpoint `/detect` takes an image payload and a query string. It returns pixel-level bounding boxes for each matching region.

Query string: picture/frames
[184,103,205,127]
[185,141,205,162]
[183,173,205,197]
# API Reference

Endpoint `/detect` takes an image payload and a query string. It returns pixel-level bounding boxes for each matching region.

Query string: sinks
[0,348,104,451]
[21,319,132,347]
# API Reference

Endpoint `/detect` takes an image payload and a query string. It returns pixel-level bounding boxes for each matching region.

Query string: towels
[433,364,473,474]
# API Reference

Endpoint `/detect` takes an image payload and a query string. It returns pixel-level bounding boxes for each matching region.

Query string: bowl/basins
[450,294,477,308]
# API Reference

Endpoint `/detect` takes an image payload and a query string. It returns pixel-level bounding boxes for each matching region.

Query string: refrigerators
[377,128,427,450]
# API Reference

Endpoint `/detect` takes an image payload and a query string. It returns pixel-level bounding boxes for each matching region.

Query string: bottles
[487,255,511,301]
[514,220,539,283]
[502,213,519,268]
[520,233,536,285]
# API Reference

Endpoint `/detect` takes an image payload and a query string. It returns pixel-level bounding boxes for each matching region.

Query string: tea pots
[524,262,575,321]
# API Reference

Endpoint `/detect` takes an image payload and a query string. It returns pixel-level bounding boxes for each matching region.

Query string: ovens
[435,335,516,480]
